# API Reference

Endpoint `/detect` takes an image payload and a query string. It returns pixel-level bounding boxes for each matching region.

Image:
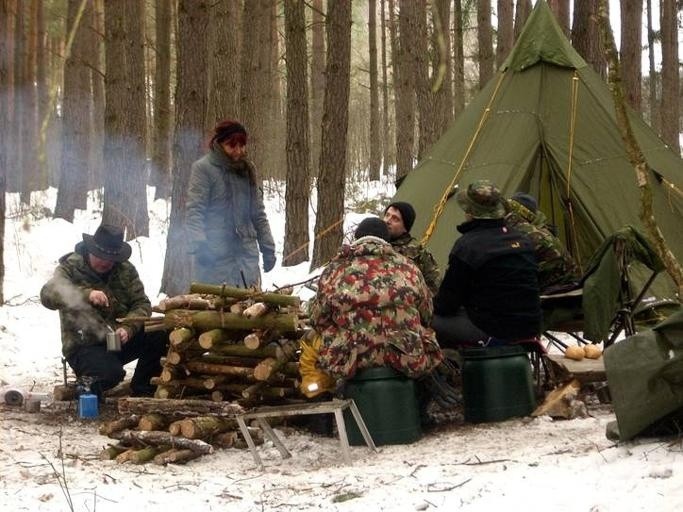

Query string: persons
[509,191,580,294]
[306,216,444,394]
[383,202,443,295]
[183,120,278,290]
[39,223,168,401]
[428,178,544,346]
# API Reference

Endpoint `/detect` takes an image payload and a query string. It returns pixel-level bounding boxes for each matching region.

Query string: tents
[392,0,683,344]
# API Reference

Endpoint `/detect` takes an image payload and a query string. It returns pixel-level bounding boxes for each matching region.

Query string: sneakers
[129,384,157,396]
[76,383,105,402]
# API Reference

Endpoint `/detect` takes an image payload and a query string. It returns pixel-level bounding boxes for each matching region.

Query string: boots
[286,391,333,435]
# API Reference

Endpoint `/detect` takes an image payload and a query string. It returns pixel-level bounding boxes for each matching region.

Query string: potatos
[566,342,602,361]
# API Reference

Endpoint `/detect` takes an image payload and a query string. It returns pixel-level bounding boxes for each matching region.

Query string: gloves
[262,250,276,273]
[189,241,215,265]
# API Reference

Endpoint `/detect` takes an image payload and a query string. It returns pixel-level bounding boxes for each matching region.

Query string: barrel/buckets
[462,346,536,424]
[345,366,423,443]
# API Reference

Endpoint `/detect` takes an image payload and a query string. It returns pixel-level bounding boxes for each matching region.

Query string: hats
[354,217,387,239]
[386,201,416,232]
[506,191,536,222]
[458,179,508,219]
[83,224,132,262]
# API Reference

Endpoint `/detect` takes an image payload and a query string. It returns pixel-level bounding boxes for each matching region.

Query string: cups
[106,332,121,352]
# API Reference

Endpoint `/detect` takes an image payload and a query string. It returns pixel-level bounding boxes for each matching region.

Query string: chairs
[539,223,635,354]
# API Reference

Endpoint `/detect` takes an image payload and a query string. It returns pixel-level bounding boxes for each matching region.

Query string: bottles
[77,386,98,420]
